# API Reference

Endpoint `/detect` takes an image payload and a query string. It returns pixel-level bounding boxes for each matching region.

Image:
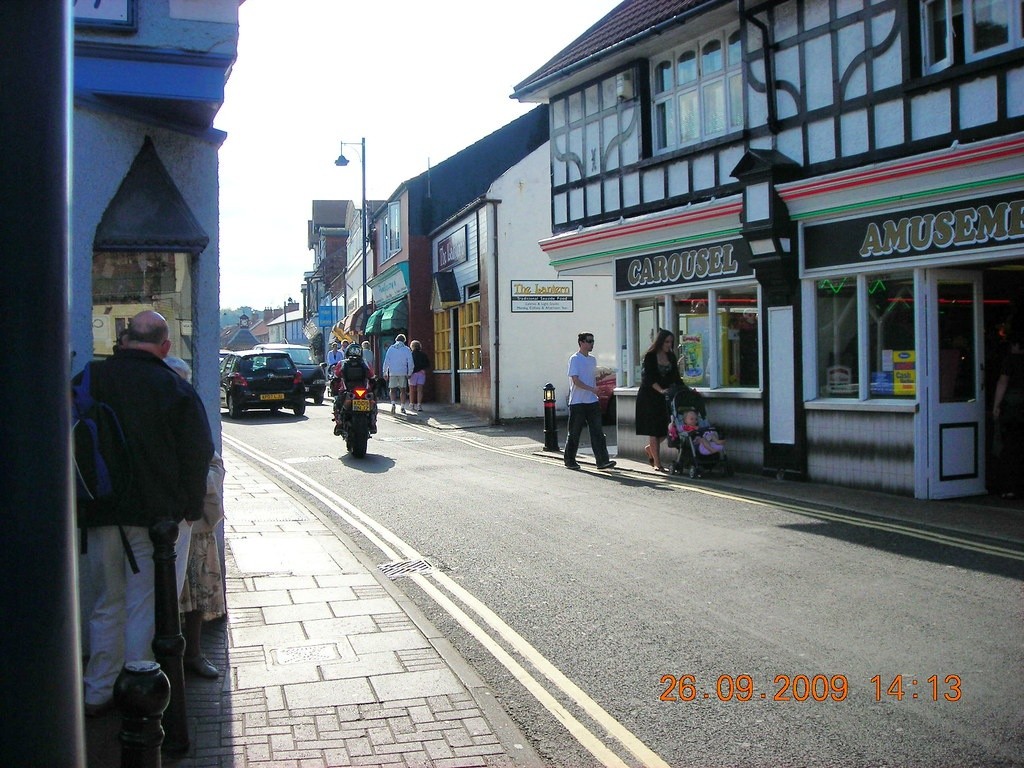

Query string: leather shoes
[183,652,219,679]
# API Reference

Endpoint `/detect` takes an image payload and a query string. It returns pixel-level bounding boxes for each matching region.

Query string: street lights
[283,296,294,343]
[335,137,370,322]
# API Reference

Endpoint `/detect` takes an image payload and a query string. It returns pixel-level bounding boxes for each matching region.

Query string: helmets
[345,344,364,358]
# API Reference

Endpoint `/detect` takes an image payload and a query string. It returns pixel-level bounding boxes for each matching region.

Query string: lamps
[542,383,556,403]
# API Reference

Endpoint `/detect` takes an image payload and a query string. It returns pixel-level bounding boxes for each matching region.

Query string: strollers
[664,387,730,481]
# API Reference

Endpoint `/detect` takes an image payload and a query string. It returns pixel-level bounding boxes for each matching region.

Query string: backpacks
[72,360,132,509]
[342,357,366,392]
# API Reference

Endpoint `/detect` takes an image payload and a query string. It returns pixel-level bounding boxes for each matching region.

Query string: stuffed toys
[668,415,678,442]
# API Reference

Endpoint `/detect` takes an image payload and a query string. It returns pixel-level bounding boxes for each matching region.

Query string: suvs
[252,343,327,405]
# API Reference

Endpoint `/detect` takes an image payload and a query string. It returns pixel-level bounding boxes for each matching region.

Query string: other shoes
[565,461,580,469]
[597,461,616,469]
[409,404,422,411]
[401,407,406,413]
[85,699,116,717]
[391,404,395,413]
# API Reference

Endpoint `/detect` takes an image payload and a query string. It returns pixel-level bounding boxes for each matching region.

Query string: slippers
[647,453,655,467]
[654,465,665,472]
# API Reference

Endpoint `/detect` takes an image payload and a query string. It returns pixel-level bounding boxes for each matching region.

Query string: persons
[993,329,1024,500]
[940,332,974,402]
[70,311,214,718]
[163,357,227,677]
[564,333,617,470]
[407,340,429,411]
[635,330,683,472]
[382,334,414,414]
[680,410,725,453]
[313,339,378,436]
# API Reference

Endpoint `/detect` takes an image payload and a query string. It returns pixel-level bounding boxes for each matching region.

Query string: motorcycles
[330,377,377,457]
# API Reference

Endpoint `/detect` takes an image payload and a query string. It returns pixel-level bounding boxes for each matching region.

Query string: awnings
[365,308,386,335]
[381,299,408,332]
[328,304,372,345]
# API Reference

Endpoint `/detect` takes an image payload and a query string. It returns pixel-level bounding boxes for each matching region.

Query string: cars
[565,369,617,426]
[219,348,305,419]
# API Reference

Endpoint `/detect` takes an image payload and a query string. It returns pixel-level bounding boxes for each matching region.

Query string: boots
[369,413,377,434]
[334,409,343,429]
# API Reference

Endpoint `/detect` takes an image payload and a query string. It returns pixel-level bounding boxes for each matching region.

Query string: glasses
[586,339,594,343]
